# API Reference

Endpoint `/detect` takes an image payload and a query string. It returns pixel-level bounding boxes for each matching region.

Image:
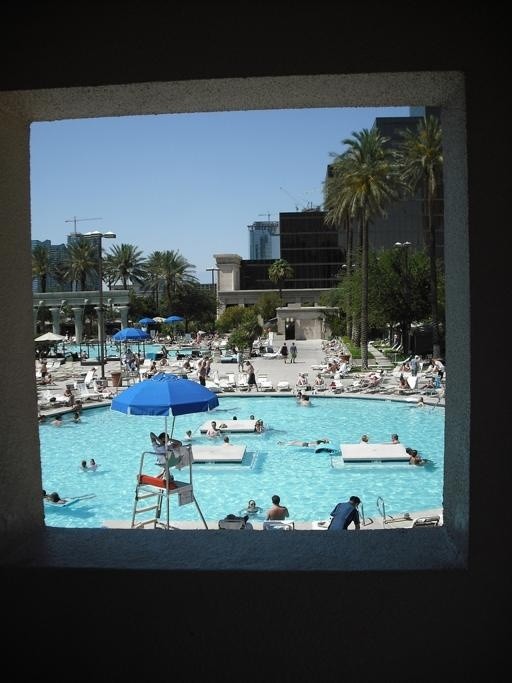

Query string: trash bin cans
[111,372,122,387]
[340,356,349,365]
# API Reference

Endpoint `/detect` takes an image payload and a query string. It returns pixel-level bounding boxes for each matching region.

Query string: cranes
[258,211,274,220]
[65,217,102,233]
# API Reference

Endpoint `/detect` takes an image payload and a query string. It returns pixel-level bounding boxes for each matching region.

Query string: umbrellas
[112,327,150,350]
[139,316,184,333]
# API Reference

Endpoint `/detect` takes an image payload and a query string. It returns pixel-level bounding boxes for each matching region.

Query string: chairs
[204,372,440,400]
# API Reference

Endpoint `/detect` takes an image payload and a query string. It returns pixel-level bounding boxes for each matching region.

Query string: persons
[240,500,262,517]
[266,495,289,520]
[327,496,360,530]
[42,490,96,507]
[34,333,446,473]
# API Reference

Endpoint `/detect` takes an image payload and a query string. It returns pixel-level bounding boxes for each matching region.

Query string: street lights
[394,241,411,357]
[206,267,220,335]
[84,231,116,379]
[341,264,358,339]
